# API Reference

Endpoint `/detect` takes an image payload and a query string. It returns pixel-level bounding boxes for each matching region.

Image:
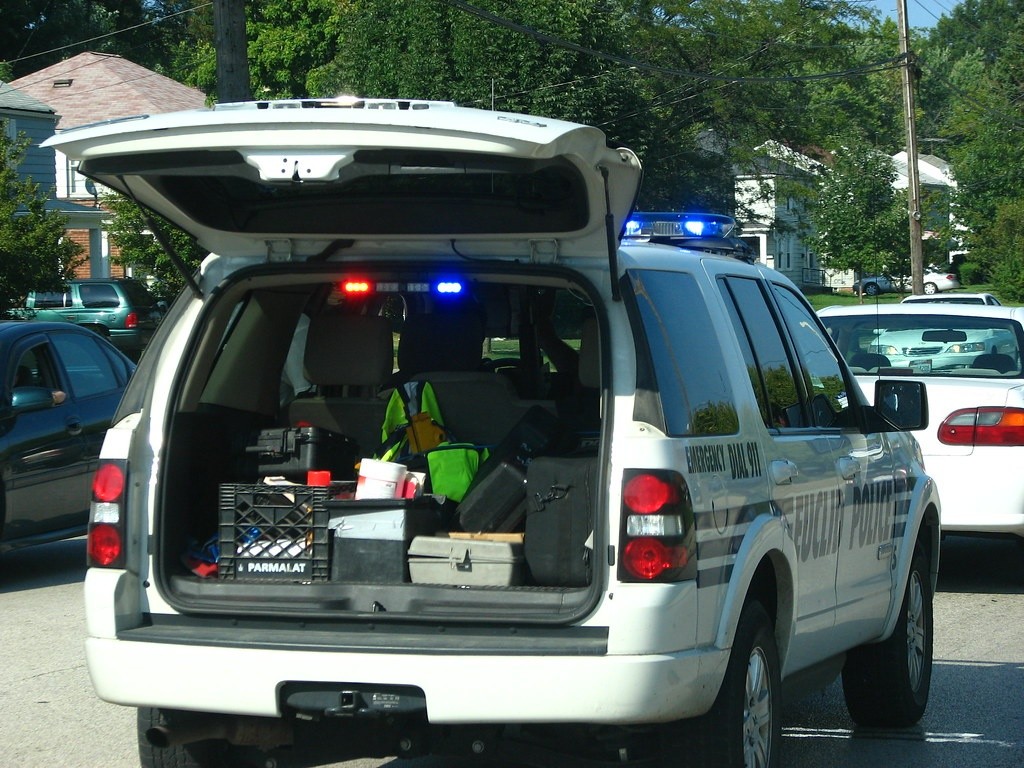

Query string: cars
[796,303,1024,544]
[851,266,959,296]
[0,321,140,576]
[864,292,1021,374]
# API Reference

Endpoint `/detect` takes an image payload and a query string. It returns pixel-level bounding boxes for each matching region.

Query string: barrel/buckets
[354,457,407,500]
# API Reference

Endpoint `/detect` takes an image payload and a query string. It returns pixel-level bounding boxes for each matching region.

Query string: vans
[36,94,945,764]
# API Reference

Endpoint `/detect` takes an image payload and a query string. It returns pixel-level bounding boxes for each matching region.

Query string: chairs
[392,316,485,387]
[288,317,393,449]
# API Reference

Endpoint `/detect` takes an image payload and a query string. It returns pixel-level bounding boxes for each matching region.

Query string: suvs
[0,276,160,354]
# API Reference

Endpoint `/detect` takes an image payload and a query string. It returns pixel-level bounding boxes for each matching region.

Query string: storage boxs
[219,482,331,582]
[322,496,447,583]
[407,535,521,587]
[247,428,352,483]
[456,464,528,534]
[526,457,596,586]
